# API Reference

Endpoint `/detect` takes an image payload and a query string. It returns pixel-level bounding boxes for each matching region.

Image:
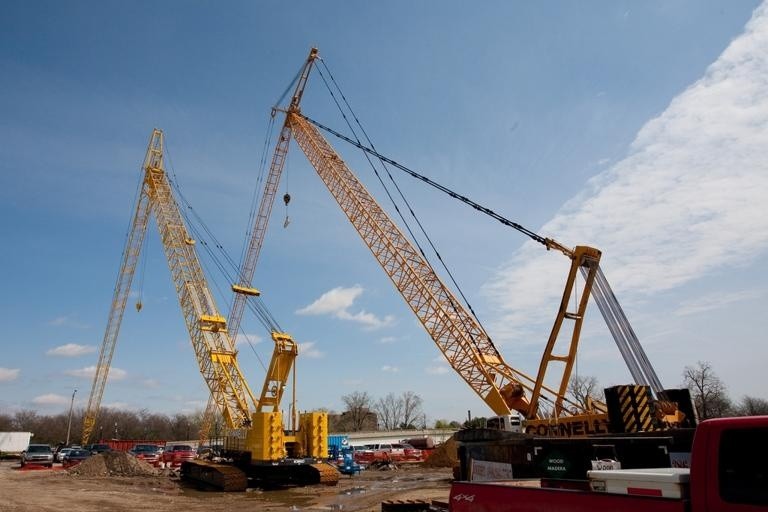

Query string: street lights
[66,388,78,444]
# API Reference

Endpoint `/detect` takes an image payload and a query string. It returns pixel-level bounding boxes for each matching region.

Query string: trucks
[449,415,768,512]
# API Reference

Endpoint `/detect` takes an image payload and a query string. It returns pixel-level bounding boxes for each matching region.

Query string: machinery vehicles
[81,128,341,492]
[198,46,700,481]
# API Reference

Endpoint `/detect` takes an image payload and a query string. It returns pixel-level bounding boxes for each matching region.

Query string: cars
[21,444,197,468]
[368,443,422,461]
